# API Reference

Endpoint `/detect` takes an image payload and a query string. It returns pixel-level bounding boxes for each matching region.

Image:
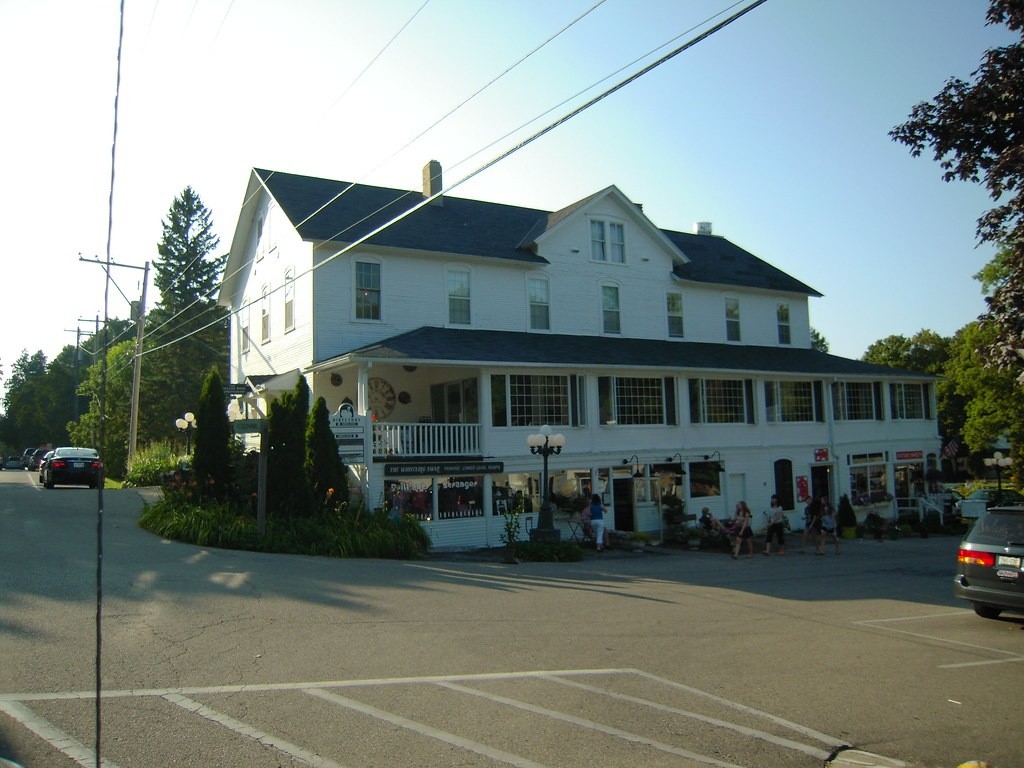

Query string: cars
[5,456,22,469]
[41,447,106,489]
[966,488,1024,508]
[40,450,53,483]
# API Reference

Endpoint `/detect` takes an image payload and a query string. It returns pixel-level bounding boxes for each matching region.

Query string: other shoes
[762,552,769,556]
[835,552,841,555]
[746,556,753,559]
[606,545,612,550]
[597,548,604,552]
[815,553,824,556]
[731,555,737,559]
[776,553,785,556]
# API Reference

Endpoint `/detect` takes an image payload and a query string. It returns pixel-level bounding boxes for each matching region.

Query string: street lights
[175,412,198,456]
[526,424,566,561]
[985,452,1013,490]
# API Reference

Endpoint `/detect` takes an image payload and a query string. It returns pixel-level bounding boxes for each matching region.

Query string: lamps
[623,455,644,478]
[704,450,725,473]
[667,453,685,475]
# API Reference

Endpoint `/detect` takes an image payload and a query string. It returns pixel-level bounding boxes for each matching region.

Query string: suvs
[954,506,1024,618]
[22,448,38,467]
[30,449,49,471]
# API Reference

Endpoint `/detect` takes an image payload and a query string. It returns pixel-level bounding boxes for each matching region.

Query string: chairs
[526,517,535,535]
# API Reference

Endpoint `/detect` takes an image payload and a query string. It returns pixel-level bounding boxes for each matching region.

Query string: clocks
[356,376,395,418]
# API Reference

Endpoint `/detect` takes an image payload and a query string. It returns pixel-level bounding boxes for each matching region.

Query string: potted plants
[920,525,928,540]
[889,522,898,539]
[869,514,884,539]
[838,493,858,541]
[497,503,521,564]
[901,523,912,537]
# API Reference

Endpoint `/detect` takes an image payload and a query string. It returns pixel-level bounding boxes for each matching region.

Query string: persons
[582,498,614,550]
[590,492,608,552]
[729,500,754,559]
[794,495,823,555]
[699,507,727,534]
[816,496,842,555]
[761,494,785,556]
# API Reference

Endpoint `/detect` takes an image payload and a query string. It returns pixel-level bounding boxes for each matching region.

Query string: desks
[566,518,591,547]
